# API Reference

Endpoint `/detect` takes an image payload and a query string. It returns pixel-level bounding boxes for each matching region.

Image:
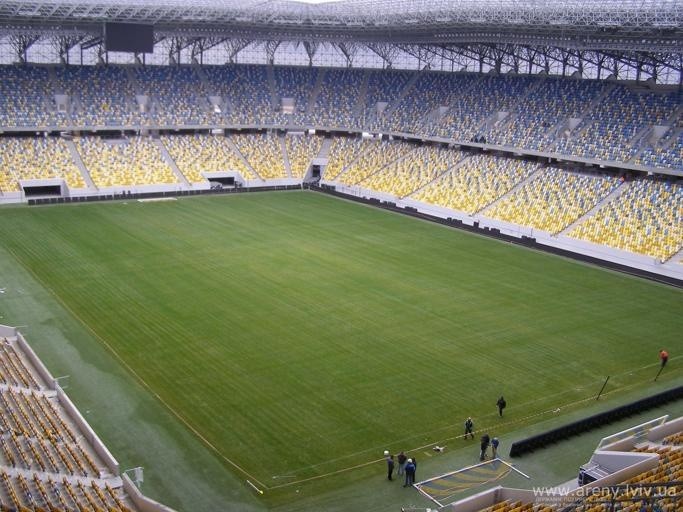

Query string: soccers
[383,450,389,455]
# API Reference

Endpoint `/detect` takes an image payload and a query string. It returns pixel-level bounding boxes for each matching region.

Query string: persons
[402,458,414,487]
[411,457,416,482]
[479,434,488,461]
[463,416,473,440]
[386,455,394,480]
[495,396,506,417]
[659,349,668,367]
[396,451,407,474]
[490,437,498,459]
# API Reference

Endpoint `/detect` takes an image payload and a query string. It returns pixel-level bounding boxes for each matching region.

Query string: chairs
[478,428,683,512]
[0,335,133,512]
